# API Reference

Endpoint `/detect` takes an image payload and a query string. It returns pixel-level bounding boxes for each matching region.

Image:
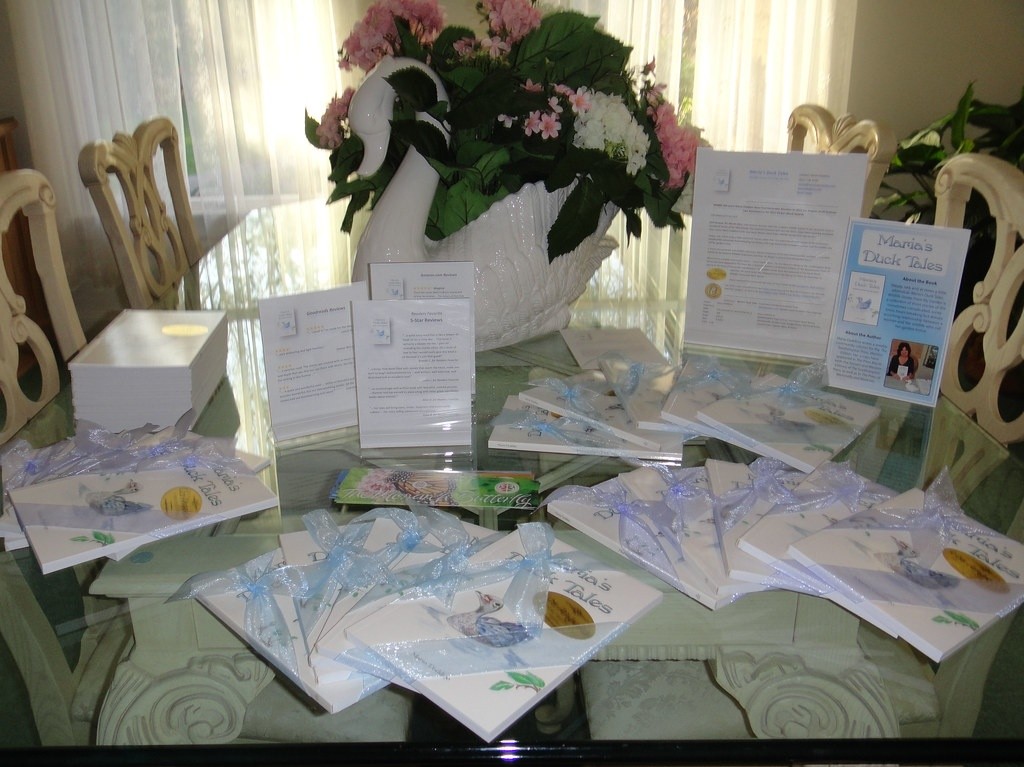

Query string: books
[193,506,666,748]
[481,353,1023,669]
[0,430,279,578]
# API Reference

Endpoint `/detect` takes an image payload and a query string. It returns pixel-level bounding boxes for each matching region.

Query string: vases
[347,54,623,354]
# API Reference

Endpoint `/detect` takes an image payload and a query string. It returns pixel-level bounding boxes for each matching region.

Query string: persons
[886,342,915,382]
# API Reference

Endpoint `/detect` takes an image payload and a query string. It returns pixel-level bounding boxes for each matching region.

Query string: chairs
[78,117,202,311]
[535,152,1024,741]
[0,168,419,744]
[785,103,895,216]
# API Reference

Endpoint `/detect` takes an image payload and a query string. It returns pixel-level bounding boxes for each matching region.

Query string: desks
[2,187,1023,767]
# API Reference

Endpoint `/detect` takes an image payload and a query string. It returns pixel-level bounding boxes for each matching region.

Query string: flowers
[304,1,716,266]
